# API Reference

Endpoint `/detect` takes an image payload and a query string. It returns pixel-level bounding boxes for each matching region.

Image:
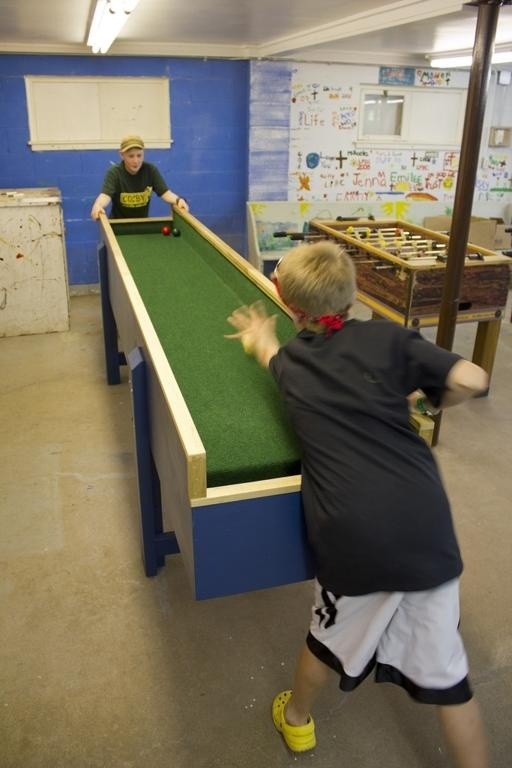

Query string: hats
[119,135,145,153]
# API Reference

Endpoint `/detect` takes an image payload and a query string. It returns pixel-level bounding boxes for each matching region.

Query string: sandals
[271,689,317,754]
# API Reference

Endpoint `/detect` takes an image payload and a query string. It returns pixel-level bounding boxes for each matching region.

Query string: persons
[90,132,191,223]
[220,239,493,768]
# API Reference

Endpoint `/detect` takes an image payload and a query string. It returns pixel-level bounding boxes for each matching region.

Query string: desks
[98,199,432,602]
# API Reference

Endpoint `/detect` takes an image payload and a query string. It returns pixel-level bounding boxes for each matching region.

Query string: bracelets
[175,196,187,205]
[415,398,442,423]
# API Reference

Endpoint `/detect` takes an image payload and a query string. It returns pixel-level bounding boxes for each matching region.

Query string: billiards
[162,225,170,235]
[172,228,181,236]
